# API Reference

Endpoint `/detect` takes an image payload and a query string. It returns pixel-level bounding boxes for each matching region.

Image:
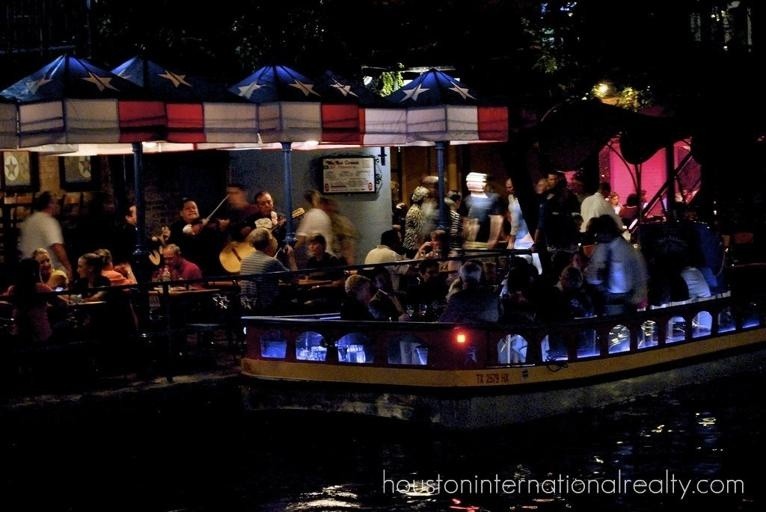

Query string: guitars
[219,208,305,273]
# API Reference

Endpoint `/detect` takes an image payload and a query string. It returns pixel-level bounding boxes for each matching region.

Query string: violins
[145,227,167,266]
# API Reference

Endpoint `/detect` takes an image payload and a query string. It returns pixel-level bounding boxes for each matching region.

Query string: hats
[411,186,430,203]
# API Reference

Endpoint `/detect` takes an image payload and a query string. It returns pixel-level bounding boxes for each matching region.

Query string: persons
[1,169,717,365]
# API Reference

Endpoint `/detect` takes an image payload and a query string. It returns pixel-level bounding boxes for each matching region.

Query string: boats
[236,309,766,446]
